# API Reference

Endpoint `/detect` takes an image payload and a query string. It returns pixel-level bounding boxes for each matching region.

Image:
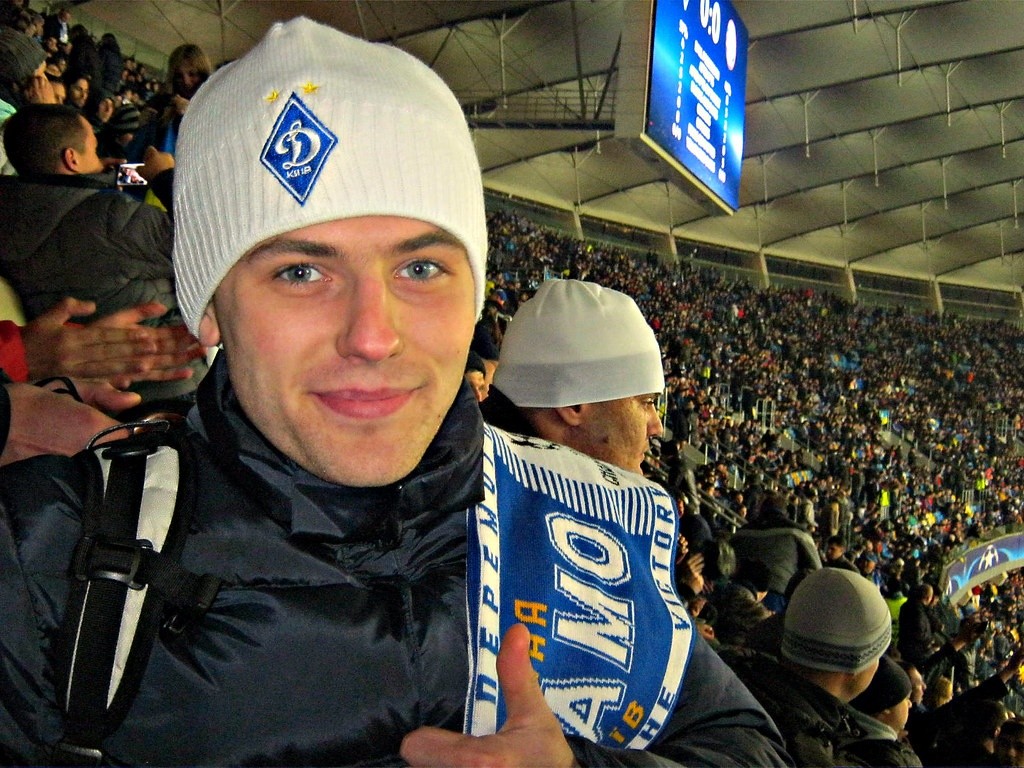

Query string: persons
[0,0,1024,768]
[841,654,923,768]
[717,567,898,768]
[0,16,796,768]
[0,104,178,326]
[463,352,487,404]
[484,277,666,476]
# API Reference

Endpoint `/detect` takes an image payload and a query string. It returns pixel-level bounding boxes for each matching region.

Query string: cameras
[116,162,150,186]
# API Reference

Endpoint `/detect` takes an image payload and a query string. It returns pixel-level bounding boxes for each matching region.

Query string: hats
[491,277,666,411]
[854,653,914,719]
[169,14,493,341]
[780,566,894,677]
[0,26,50,97]
